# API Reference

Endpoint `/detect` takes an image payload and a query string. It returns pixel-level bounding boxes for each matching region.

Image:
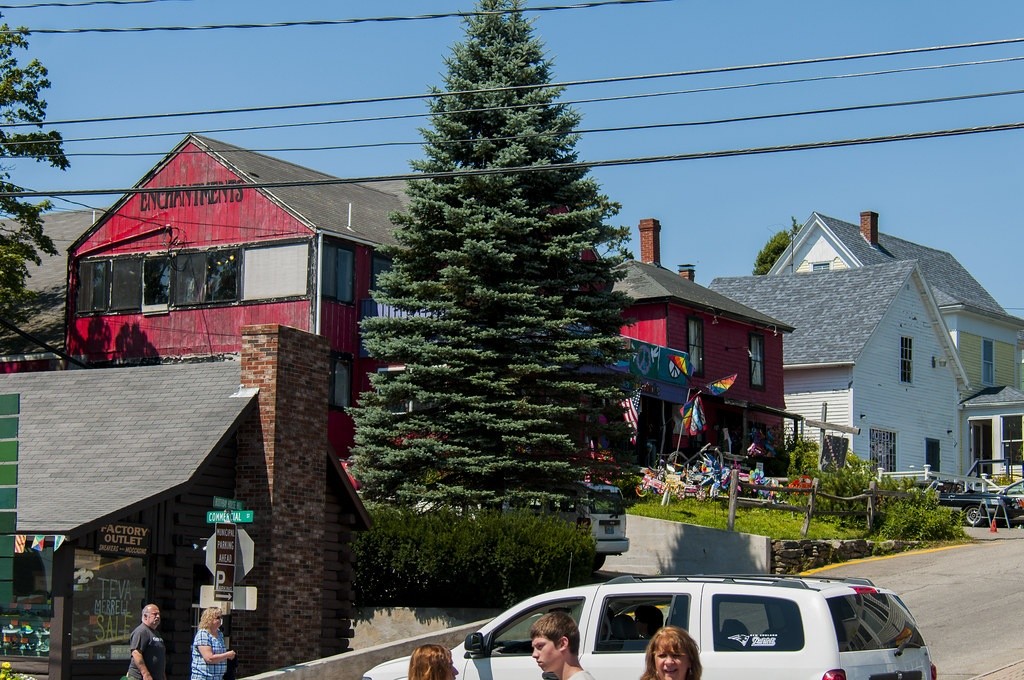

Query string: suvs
[361,575,936,680]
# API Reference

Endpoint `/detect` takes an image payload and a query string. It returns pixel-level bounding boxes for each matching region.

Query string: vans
[416,481,630,570]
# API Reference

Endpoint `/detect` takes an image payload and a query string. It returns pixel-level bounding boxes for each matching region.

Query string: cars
[913,480,1022,526]
[996,479,1024,509]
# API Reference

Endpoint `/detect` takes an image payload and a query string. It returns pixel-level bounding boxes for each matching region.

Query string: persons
[126,604,166,680]
[639,626,702,680]
[408,644,459,680]
[191,607,236,680]
[635,605,663,639]
[530,612,595,680]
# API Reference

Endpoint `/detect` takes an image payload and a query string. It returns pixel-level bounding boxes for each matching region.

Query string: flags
[53,535,65,552]
[31,536,45,551]
[14,535,26,553]
[680,394,708,437]
[620,387,642,445]
